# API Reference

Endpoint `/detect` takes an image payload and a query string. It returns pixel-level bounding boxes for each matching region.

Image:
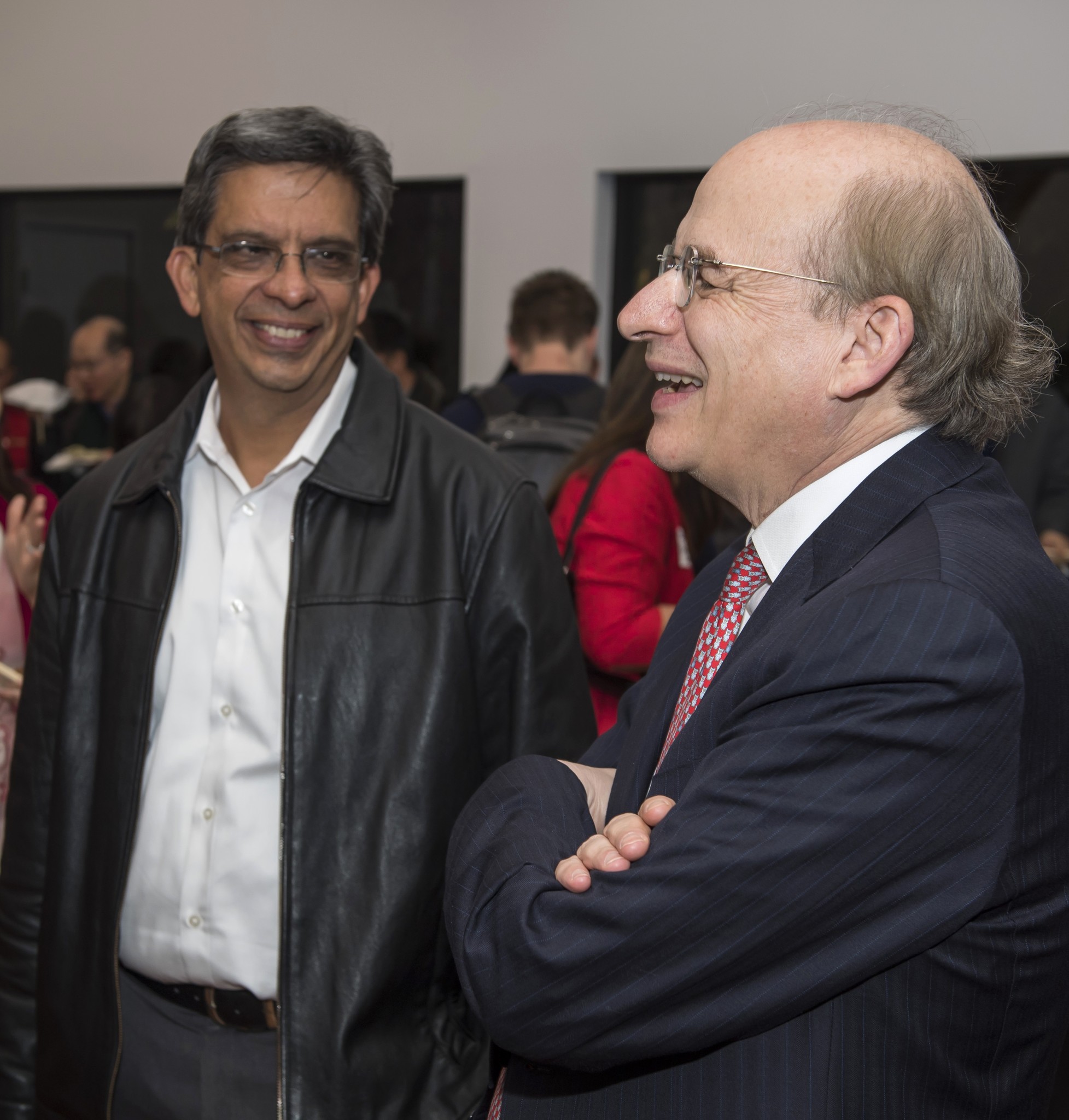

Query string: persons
[116,371,183,458]
[3,104,595,1120]
[151,336,211,389]
[1,432,59,637]
[0,535,22,819]
[49,304,142,454]
[438,102,1069,1117]
[440,271,611,503]
[0,336,40,472]
[543,318,705,751]
[357,304,450,416]
[976,381,1069,579]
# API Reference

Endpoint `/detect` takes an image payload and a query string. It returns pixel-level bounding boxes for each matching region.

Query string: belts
[120,963,280,1031]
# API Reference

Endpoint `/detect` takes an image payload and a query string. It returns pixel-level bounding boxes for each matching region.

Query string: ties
[487,546,769,1114]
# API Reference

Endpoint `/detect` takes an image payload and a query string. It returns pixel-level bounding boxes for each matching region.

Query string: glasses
[182,241,370,284]
[657,245,844,311]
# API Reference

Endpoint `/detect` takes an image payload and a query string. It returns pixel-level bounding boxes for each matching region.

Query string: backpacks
[478,414,600,502]
